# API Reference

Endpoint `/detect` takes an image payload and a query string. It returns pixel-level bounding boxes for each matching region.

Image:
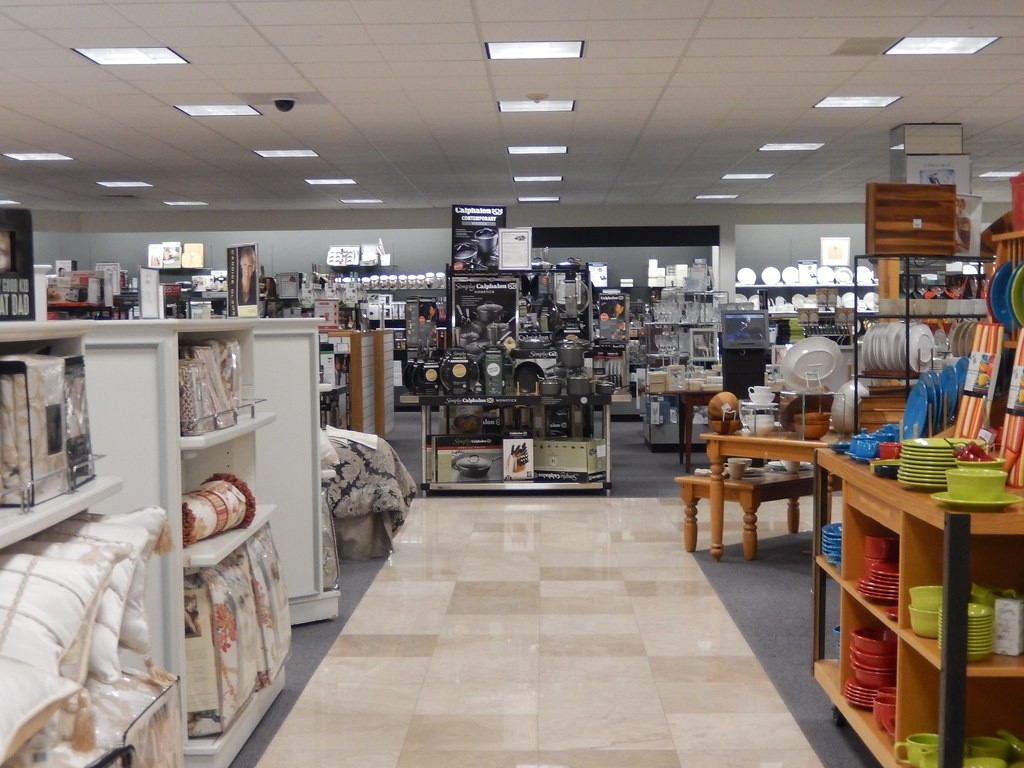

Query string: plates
[819,520,1017,709]
[722,457,812,476]
[781,321,935,392]
[897,320,1023,510]
[985,261,1023,336]
[735,267,879,317]
[827,442,870,463]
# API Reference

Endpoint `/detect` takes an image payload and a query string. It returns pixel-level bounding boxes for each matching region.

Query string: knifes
[511,443,527,455]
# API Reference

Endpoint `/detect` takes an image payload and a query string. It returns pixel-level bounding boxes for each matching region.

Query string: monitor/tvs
[721,310,770,349]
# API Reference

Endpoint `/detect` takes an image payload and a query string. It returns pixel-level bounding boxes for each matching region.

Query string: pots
[514,359,556,394]
[540,335,615,396]
[531,257,580,272]
[453,455,503,478]
[450,414,483,432]
[455,301,516,350]
[403,347,485,397]
[452,227,498,273]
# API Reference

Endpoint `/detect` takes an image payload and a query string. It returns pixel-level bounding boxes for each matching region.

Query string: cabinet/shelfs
[0,182,1024,768]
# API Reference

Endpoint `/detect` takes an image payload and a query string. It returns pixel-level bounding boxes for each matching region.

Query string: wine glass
[738,398,753,434]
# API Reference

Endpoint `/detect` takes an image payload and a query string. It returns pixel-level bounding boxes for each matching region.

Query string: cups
[644,299,722,376]
[899,272,991,315]
[779,460,800,473]
[954,457,1005,471]
[748,386,771,394]
[723,462,745,481]
[871,686,1024,768]
[945,468,1008,501]
[849,423,902,461]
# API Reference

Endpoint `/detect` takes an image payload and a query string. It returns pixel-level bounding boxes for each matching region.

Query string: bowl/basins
[727,458,752,472]
[707,381,869,439]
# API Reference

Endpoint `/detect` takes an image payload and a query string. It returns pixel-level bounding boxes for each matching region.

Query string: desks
[699,427,847,563]
[675,466,815,558]
[675,387,780,474]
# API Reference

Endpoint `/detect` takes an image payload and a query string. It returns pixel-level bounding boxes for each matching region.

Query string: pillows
[0,665,83,768]
[30,519,150,683]
[66,505,167,664]
[0,541,129,685]
[0,553,111,675]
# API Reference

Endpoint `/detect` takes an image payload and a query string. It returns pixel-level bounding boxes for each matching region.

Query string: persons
[151,256,160,266]
[163,247,180,268]
[237,246,256,306]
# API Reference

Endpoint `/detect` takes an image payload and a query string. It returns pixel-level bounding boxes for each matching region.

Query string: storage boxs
[534,436,606,482]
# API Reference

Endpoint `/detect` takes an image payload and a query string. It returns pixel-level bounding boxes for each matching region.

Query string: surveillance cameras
[274,99,295,113]
